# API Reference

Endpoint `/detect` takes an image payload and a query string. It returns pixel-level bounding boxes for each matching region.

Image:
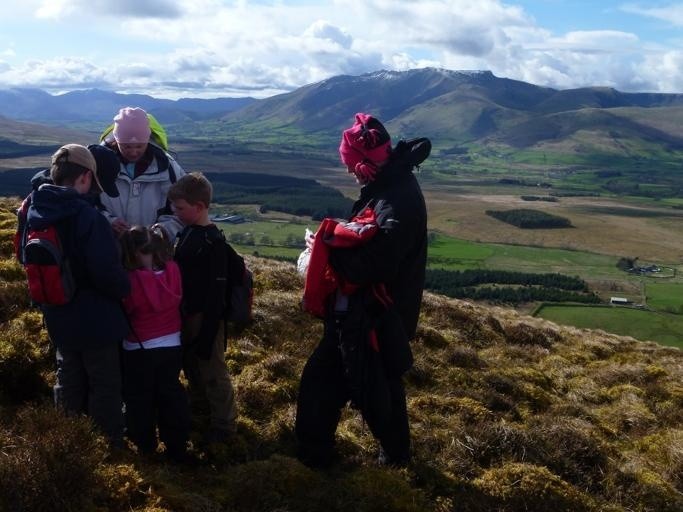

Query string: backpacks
[180,229,254,338]
[20,194,88,310]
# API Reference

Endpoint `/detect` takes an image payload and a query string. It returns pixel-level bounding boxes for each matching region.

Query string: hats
[87,144,121,198]
[51,143,104,195]
[338,112,392,165]
[112,107,151,144]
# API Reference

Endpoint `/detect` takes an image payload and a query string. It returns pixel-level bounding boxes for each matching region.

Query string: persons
[119,224,189,465]
[167,170,240,448]
[20,144,137,465]
[270,112,433,488]
[12,144,121,268]
[85,107,191,427]
